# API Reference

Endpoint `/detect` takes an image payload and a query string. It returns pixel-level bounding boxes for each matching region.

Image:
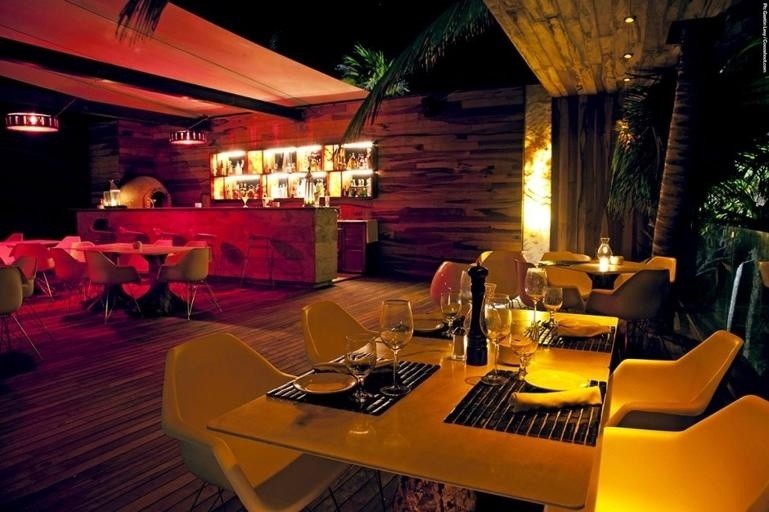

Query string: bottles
[262,195,267,207]
[227,177,372,199]
[596,237,613,263]
[218,148,371,175]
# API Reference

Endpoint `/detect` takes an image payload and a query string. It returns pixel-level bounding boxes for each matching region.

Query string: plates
[408,317,443,332]
[292,372,357,394]
[523,369,589,392]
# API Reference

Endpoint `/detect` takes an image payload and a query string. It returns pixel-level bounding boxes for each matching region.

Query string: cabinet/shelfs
[337,217,380,274]
[210,140,378,201]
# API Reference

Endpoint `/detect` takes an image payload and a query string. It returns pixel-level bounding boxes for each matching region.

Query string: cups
[484,282,496,300]
[612,256,624,265]
[195,203,202,208]
[325,196,330,207]
[102,189,121,206]
[272,202,281,208]
[498,341,531,365]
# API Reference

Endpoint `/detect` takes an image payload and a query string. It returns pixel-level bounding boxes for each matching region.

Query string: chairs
[607,331,743,424]
[2,267,44,361]
[156,246,223,320]
[83,250,142,323]
[304,302,382,368]
[163,331,351,512]
[543,396,767,510]
[1,232,219,299]
[432,248,679,353]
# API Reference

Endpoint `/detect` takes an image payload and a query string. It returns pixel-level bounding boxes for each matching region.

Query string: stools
[241,235,277,288]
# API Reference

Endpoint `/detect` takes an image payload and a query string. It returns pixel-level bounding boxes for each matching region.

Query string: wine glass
[242,198,248,209]
[438,291,464,339]
[525,267,548,327]
[346,329,378,405]
[481,295,511,387]
[541,286,563,326]
[378,297,413,399]
[511,319,542,383]
[150,199,156,208]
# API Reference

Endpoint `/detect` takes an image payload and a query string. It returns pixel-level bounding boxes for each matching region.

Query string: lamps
[170,130,208,145]
[6,113,61,134]
